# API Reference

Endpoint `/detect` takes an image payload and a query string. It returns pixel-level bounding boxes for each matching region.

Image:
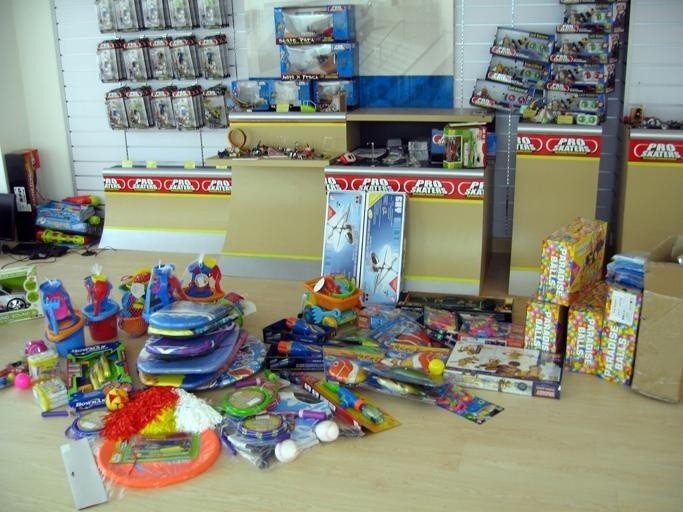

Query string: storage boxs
[439,337,566,403]
[536,214,609,312]
[562,279,611,376]
[35,212,100,238]
[223,1,365,117]
[0,262,45,324]
[521,292,570,359]
[463,0,632,125]
[459,122,491,171]
[317,185,369,291]
[630,236,682,405]
[35,199,96,223]
[353,189,410,311]
[597,279,644,389]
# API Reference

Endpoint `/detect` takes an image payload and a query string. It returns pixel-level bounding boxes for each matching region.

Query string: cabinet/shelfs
[217,109,494,297]
